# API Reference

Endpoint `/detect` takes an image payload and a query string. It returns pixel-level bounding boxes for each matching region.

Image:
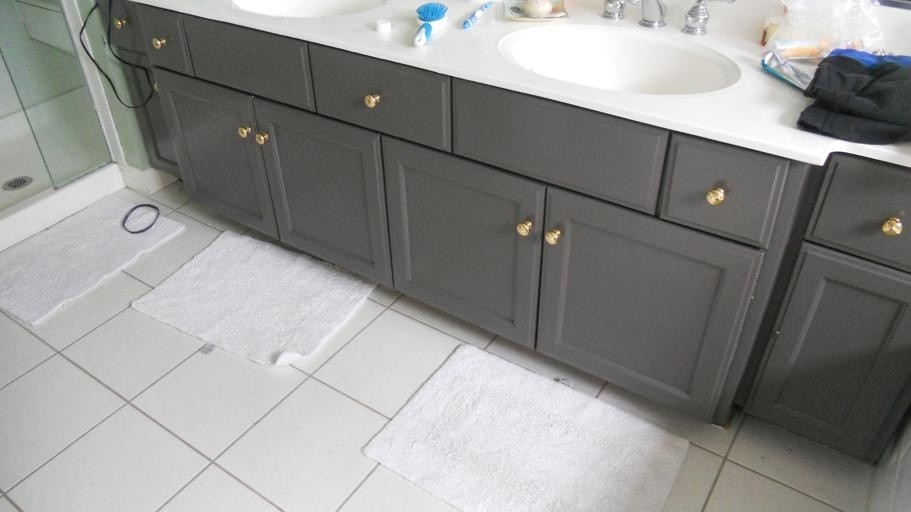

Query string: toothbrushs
[462,1,493,30]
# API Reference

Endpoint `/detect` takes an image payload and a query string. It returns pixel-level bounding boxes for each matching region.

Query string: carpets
[363,342,693,511]
[127,226,380,369]
[0,184,184,330]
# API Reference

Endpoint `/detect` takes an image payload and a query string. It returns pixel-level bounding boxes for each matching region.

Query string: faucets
[637,0,667,29]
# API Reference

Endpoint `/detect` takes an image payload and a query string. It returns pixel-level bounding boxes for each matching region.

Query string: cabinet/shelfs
[656,128,791,250]
[302,43,451,161]
[450,78,669,217]
[132,3,195,79]
[378,131,769,432]
[99,0,182,182]
[178,12,317,115]
[150,63,396,293]
[738,143,909,472]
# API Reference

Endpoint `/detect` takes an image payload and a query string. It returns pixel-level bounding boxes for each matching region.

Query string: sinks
[497,21,743,97]
[229,0,389,20]
